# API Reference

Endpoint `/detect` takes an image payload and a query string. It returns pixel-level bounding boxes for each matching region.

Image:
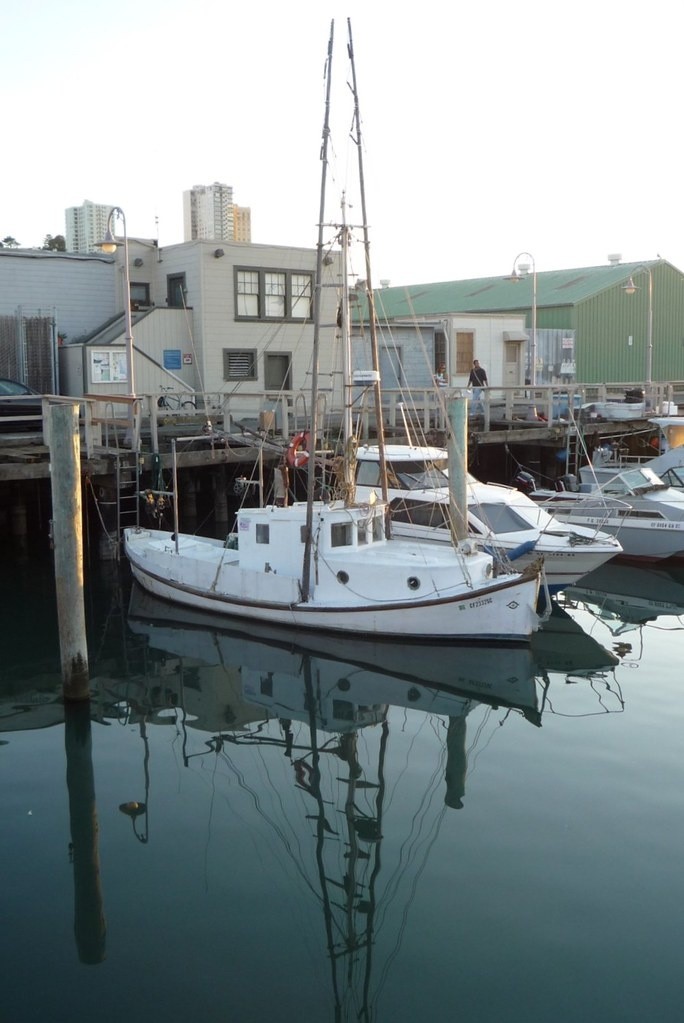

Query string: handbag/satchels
[437,379,448,385]
[480,391,484,400]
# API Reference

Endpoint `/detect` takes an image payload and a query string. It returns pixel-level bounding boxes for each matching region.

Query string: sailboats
[126,583,549,1021]
[117,14,547,649]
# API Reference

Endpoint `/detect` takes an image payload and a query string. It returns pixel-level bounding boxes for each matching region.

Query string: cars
[0,380,55,430]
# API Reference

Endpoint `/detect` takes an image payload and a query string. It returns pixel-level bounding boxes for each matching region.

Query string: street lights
[620,263,653,402]
[505,252,538,425]
[94,207,138,449]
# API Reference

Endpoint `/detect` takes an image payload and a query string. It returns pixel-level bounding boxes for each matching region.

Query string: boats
[534,559,684,715]
[341,438,631,590]
[529,440,683,565]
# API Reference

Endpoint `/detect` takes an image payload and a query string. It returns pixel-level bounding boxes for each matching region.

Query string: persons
[436,364,449,388]
[466,360,489,416]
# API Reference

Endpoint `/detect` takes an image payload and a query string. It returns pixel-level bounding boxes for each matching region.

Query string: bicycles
[156,386,199,413]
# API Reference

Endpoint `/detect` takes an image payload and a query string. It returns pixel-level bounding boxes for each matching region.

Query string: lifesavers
[287,431,310,467]
